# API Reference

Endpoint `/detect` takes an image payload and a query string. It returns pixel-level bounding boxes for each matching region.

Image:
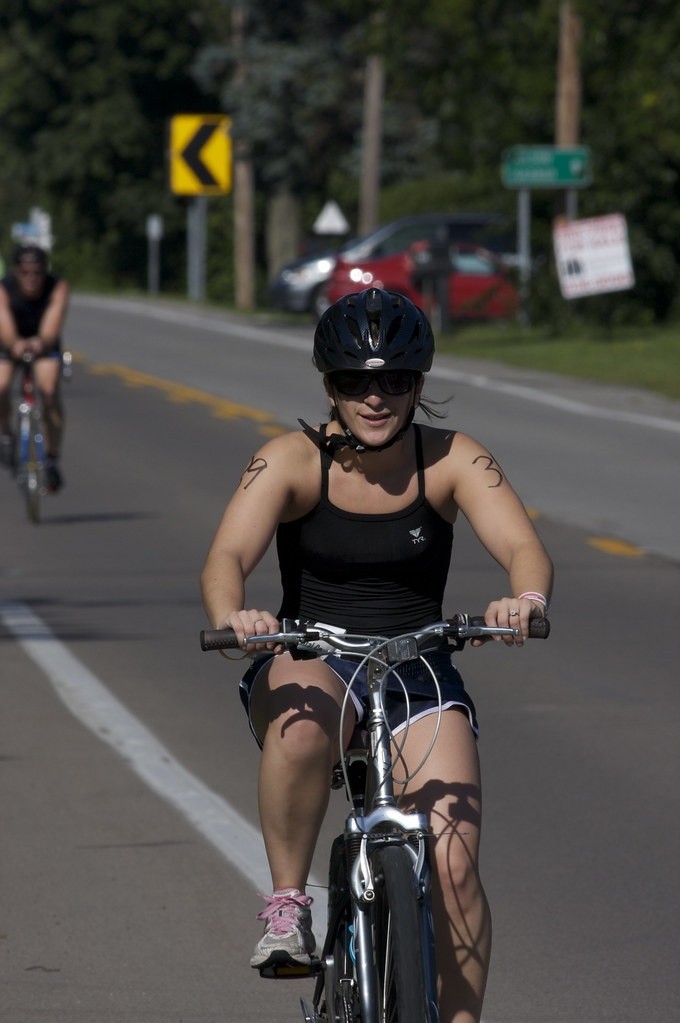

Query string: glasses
[330,369,421,398]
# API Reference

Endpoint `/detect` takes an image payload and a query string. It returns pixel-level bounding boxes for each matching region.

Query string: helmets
[312,287,435,378]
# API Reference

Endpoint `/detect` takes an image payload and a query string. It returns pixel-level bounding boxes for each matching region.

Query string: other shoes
[42,455,61,493]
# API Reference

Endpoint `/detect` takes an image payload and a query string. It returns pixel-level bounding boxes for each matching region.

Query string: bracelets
[518,591,548,611]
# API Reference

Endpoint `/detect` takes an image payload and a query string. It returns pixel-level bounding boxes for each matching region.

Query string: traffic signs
[501,143,601,194]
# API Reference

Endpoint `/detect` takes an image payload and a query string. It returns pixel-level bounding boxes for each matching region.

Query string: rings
[254,618,264,624]
[508,611,520,616]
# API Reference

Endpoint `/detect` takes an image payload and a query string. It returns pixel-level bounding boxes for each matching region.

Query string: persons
[0,243,72,496]
[200,287,555,1023]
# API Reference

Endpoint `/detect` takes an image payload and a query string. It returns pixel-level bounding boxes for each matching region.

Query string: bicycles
[0,340,73,524]
[197,615,552,1023]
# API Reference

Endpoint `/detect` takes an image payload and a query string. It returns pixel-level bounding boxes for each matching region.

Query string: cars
[326,236,521,323]
[281,208,545,326]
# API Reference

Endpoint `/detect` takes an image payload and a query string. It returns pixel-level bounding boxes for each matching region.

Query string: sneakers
[250,888,316,969]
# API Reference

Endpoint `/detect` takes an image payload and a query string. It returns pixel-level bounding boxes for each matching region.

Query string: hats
[13,246,46,266]
